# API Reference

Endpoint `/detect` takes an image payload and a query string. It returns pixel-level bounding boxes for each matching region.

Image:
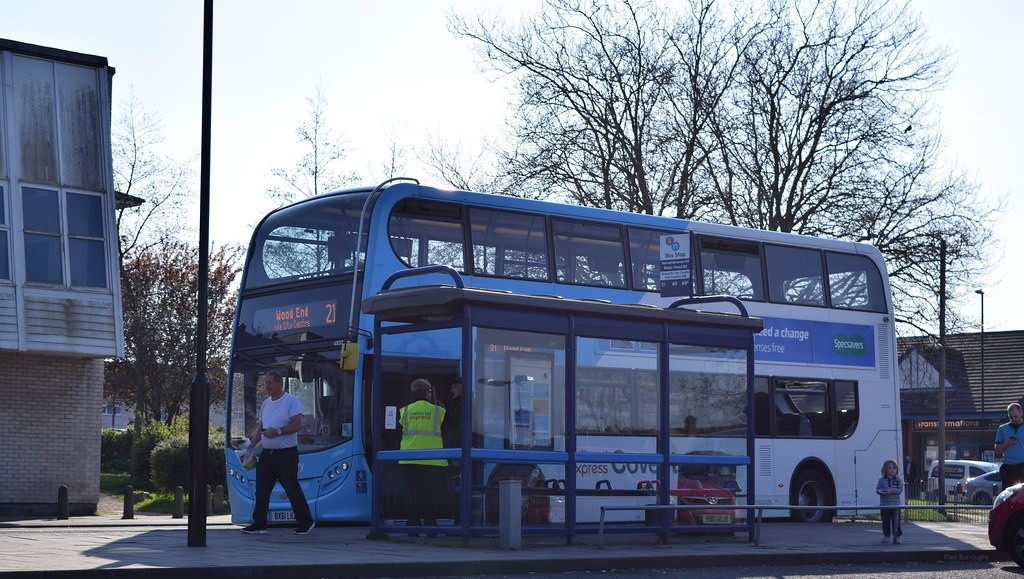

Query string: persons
[995,404,1024,490]
[874,461,904,544]
[396,378,449,536]
[240,371,315,535]
[440,377,468,448]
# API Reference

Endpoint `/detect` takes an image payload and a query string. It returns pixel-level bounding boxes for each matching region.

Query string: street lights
[973,289,986,458]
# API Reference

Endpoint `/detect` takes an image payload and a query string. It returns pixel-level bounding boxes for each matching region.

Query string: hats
[411,379,431,398]
[449,377,462,385]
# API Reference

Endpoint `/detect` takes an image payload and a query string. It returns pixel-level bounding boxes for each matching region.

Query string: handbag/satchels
[235,442,259,468]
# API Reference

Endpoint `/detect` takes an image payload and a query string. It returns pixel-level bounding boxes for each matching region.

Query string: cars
[967,469,1004,507]
[988,482,1024,569]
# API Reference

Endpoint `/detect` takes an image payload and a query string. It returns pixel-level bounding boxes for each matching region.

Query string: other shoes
[882,534,889,542]
[893,537,901,544]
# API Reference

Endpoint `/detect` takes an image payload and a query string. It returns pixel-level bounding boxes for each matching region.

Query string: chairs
[753,391,856,437]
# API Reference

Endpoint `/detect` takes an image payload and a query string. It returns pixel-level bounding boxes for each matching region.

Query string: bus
[223,176,910,528]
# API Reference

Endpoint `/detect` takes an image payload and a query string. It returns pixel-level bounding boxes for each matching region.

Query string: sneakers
[294,522,314,535]
[241,525,267,533]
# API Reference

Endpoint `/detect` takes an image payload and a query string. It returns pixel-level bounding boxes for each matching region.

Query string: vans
[928,459,1001,503]
